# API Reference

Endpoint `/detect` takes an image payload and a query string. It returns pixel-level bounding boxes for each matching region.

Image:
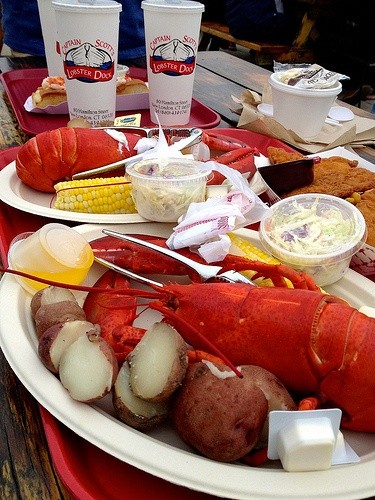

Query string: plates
[305,147,375,173]
[0,151,273,224]
[24,82,150,114]
[0,224,375,500]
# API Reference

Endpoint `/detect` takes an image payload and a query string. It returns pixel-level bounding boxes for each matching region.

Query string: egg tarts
[267,409,362,472]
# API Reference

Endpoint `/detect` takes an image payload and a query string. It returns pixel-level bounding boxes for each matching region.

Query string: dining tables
[0,50,375,500]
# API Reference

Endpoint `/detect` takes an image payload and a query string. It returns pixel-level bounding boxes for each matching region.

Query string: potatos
[31,286,297,463]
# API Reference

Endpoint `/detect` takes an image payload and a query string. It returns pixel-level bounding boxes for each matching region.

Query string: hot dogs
[33,76,149,108]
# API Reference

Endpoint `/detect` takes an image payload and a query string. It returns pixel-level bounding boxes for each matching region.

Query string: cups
[141,0,205,126]
[51,0,123,128]
[37,0,64,77]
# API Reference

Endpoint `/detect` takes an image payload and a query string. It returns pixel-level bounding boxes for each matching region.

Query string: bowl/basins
[259,194,368,287]
[8,224,94,295]
[268,72,342,140]
[125,158,214,222]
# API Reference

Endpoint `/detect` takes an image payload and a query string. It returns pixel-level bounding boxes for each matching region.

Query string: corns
[53,176,138,214]
[227,231,328,294]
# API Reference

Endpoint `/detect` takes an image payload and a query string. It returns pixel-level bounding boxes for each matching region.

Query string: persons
[0,0,375,89]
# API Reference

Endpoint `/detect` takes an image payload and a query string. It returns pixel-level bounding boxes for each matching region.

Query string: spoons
[257,104,343,127]
[327,107,354,121]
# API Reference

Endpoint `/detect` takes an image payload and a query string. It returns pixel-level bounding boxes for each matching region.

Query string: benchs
[200,22,289,57]
